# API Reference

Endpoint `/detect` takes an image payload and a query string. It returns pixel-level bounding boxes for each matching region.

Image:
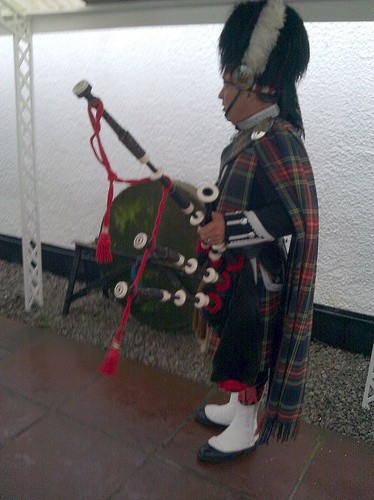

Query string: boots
[197,398,262,461]
[196,391,240,428]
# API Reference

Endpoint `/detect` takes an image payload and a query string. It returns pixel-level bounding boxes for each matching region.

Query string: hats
[219,1,310,96]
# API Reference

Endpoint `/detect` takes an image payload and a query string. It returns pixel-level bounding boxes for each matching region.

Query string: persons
[189,71,319,464]
